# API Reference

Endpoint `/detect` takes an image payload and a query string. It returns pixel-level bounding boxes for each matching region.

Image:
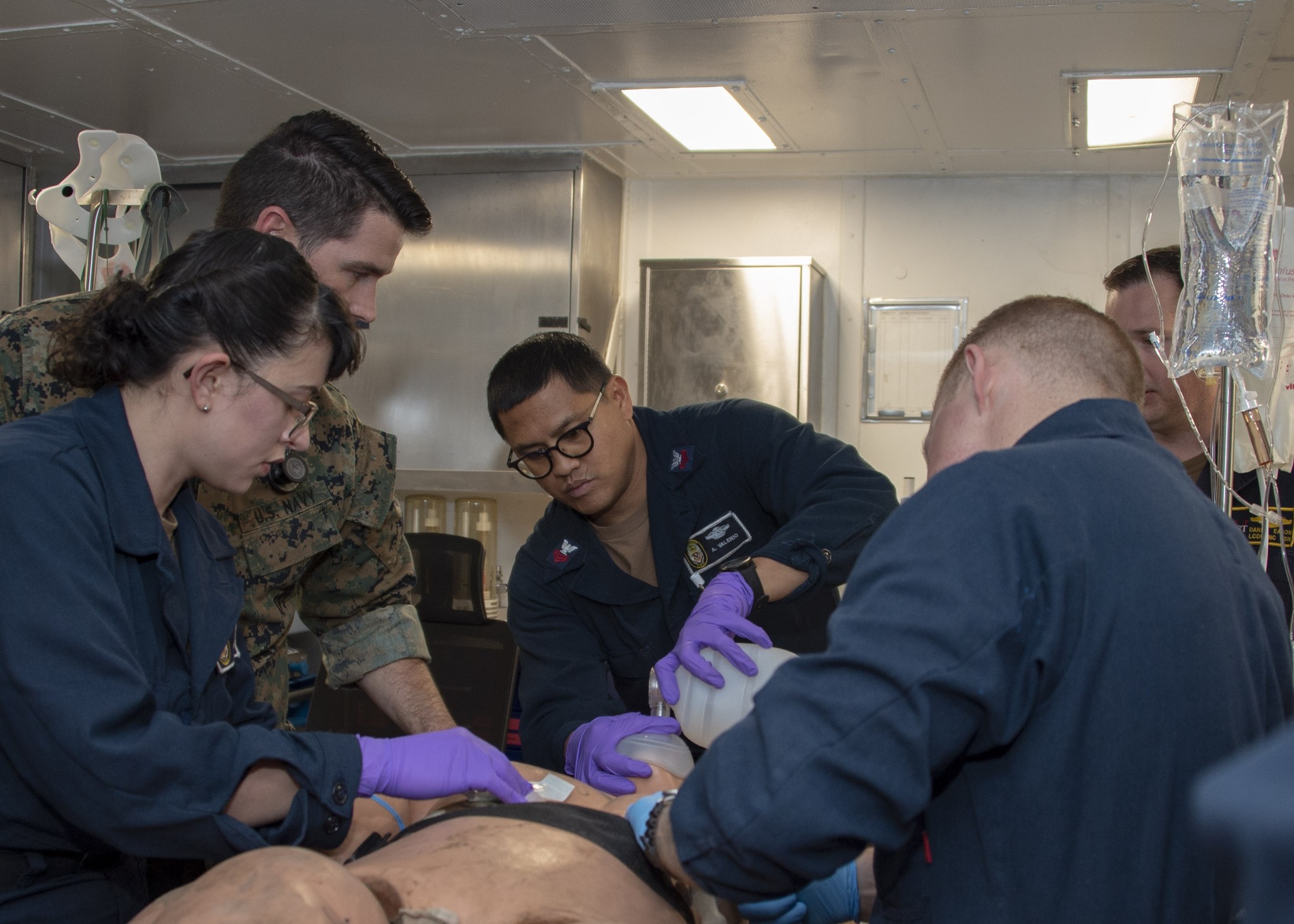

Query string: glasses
[182,356,319,441]
[507,382,611,479]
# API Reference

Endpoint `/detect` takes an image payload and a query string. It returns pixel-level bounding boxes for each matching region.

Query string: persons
[0,110,457,733]
[131,756,694,924]
[623,294,1294,924]
[1103,244,1294,614]
[487,332,900,924]
[0,224,534,924]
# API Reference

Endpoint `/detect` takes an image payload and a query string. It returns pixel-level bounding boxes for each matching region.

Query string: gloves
[562,713,680,795]
[736,861,862,924]
[355,727,532,809]
[626,788,665,846]
[653,571,774,708]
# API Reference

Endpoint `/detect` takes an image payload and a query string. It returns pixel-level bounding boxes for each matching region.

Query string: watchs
[720,556,769,610]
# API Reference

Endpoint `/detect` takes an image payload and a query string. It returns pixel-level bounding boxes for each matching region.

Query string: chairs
[304,532,521,754]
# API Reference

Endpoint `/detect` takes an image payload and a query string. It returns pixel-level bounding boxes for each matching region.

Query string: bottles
[615,732,695,778]
[648,642,799,750]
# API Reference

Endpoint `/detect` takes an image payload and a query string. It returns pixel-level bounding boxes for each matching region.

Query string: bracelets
[643,789,678,846]
